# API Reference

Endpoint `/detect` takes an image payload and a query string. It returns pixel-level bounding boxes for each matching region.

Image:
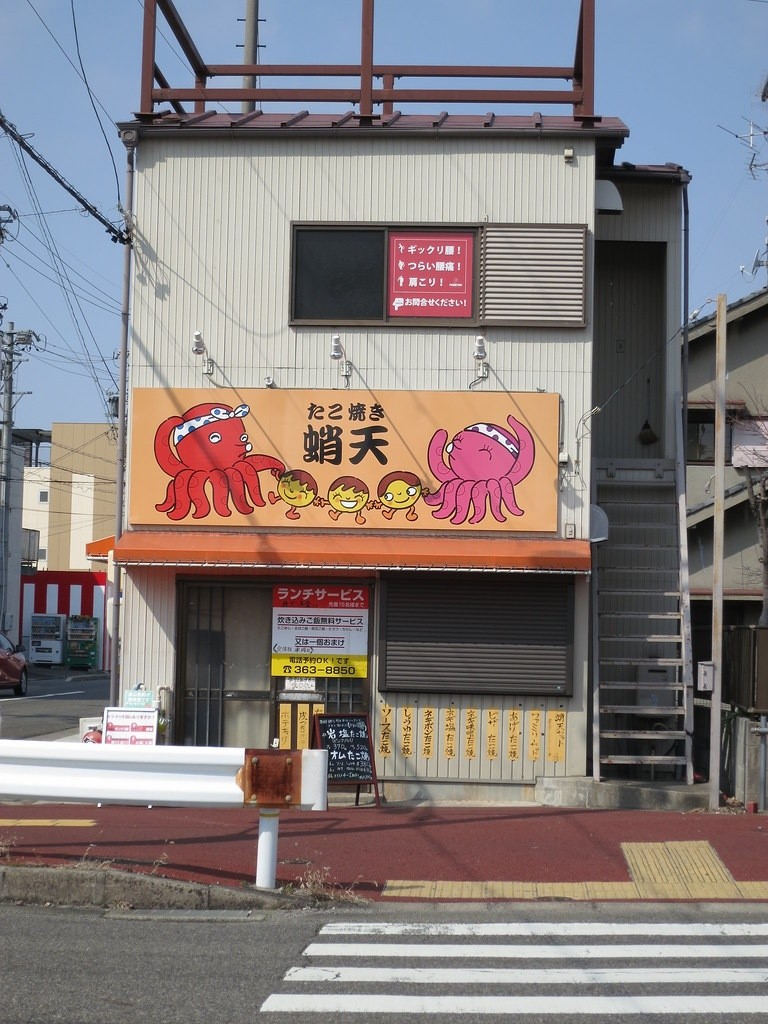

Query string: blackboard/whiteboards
[308,711,380,786]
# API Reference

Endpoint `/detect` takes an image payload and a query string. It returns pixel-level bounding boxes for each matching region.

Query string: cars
[0,632,28,696]
